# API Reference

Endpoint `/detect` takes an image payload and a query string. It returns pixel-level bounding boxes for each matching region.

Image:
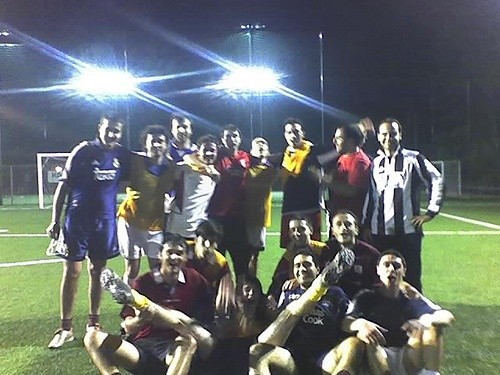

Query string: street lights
[67,66,139,151]
[239,22,269,141]
[218,62,281,137]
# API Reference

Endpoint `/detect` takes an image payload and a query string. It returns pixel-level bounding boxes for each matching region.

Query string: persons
[320,122,372,240]
[360,118,446,294]
[267,116,374,248]
[83,208,456,375]
[46,110,280,348]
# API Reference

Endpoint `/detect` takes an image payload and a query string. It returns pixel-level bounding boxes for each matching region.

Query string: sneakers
[323,247,355,286]
[86,324,102,332]
[47,328,73,348]
[100,269,134,305]
